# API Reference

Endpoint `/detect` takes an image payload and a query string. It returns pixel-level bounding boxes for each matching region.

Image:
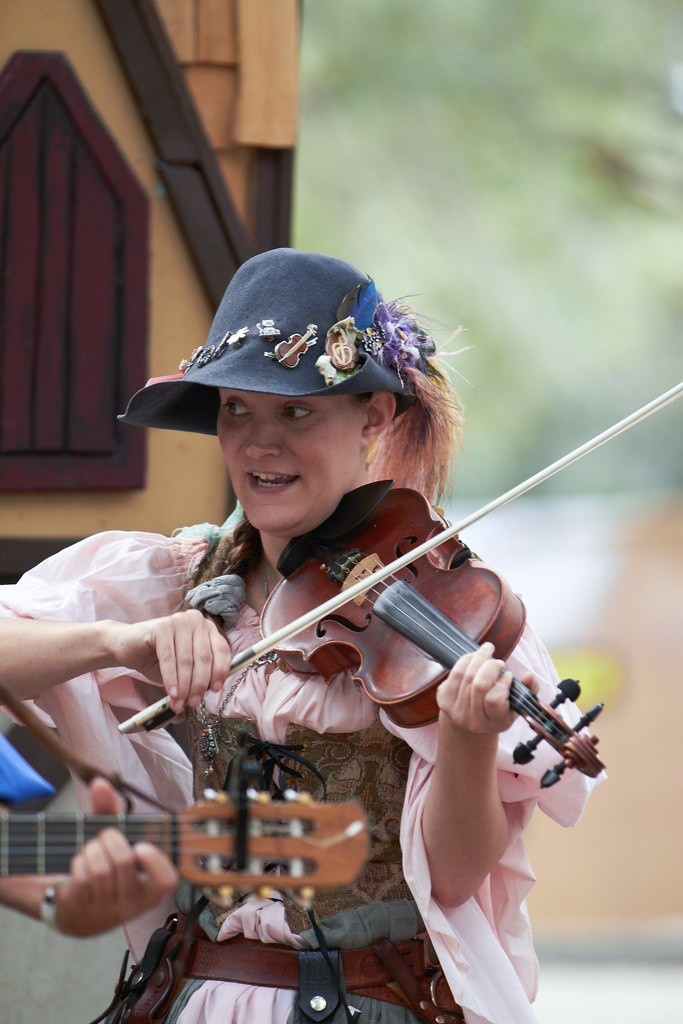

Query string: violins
[258,487,607,789]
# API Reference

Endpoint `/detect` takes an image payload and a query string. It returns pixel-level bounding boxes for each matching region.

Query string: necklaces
[260,554,276,663]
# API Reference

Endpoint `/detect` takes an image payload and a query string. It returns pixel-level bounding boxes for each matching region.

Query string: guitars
[0,789,372,905]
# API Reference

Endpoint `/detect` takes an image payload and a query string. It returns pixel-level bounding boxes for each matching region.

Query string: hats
[115,248,463,509]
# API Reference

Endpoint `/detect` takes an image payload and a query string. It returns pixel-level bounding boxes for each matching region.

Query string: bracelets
[38,882,62,935]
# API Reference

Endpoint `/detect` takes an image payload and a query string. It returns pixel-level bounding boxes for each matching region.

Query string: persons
[1,246,605,1024]
